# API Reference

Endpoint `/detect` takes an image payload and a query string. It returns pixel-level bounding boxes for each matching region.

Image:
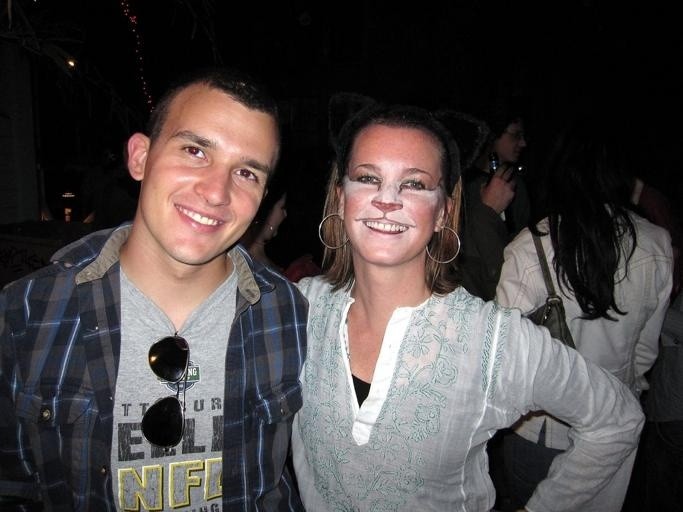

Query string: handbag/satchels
[526,225,576,348]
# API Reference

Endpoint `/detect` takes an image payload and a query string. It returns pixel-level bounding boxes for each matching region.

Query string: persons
[1,68,310,511]
[238,184,322,278]
[40,170,120,233]
[291,103,645,511]
[450,104,681,511]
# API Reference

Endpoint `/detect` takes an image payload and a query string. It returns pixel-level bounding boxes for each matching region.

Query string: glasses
[141,335,189,447]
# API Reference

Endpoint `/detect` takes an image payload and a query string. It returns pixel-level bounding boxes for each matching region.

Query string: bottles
[487,153,499,182]
